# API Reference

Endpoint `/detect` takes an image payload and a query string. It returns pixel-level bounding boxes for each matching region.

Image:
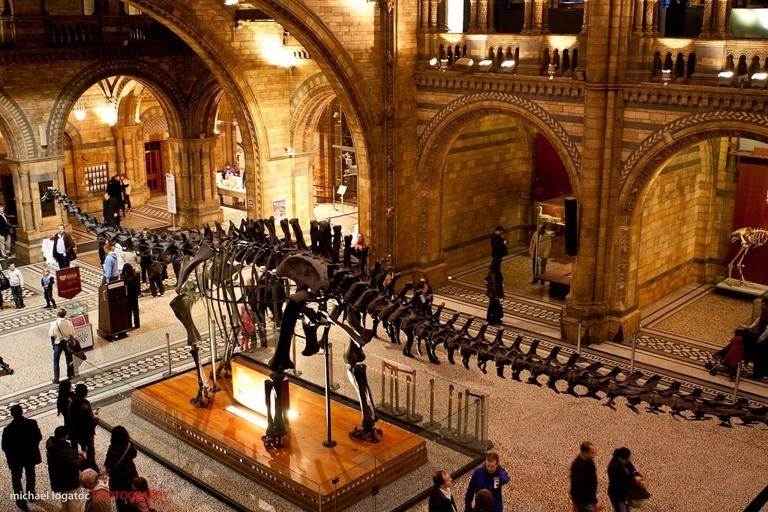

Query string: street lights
[0,278,11,291]
[66,338,81,353]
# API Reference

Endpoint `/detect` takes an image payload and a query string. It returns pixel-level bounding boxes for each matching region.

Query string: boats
[563,196,578,257]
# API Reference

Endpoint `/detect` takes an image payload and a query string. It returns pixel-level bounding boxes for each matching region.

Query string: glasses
[717,68,736,86]
[428,55,556,80]
[749,70,768,90]
[660,67,672,86]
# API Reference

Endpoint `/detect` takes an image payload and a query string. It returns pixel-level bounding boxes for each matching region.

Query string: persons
[0,175,182,511]
[483,225,508,285]
[221,162,235,180]
[570,441,601,512]
[529,223,552,290]
[484,259,505,326]
[608,446,642,511]
[351,234,433,355]
[237,265,287,351]
[429,452,509,512]
[727,336,743,381]
[735,296,768,381]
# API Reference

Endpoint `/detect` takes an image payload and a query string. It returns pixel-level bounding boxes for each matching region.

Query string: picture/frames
[216,180,246,210]
